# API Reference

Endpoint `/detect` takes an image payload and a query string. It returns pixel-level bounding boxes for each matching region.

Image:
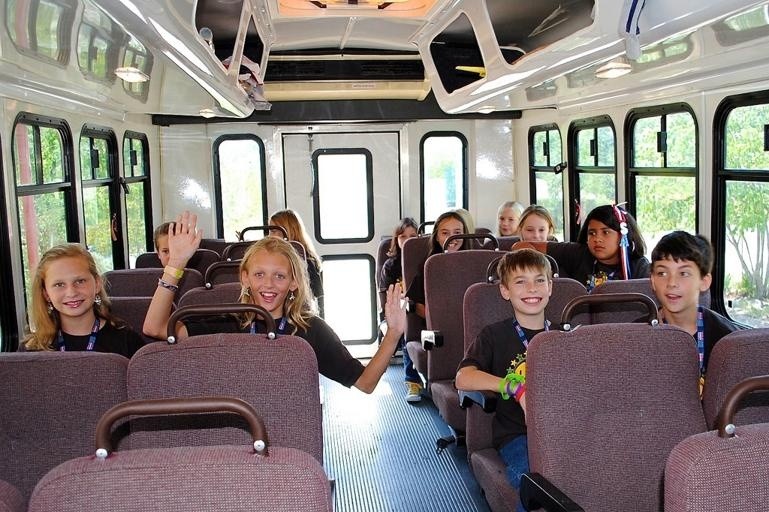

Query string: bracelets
[158,279,179,293]
[164,264,183,281]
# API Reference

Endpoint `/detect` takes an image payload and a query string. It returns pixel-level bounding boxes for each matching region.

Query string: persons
[269,210,323,321]
[380,200,554,402]
[454,247,567,511]
[141,210,410,393]
[14,243,146,360]
[630,230,743,406]
[154,221,189,270]
[570,205,653,296]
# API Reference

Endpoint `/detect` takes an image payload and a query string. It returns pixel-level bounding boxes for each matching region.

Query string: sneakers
[404,381,423,401]
